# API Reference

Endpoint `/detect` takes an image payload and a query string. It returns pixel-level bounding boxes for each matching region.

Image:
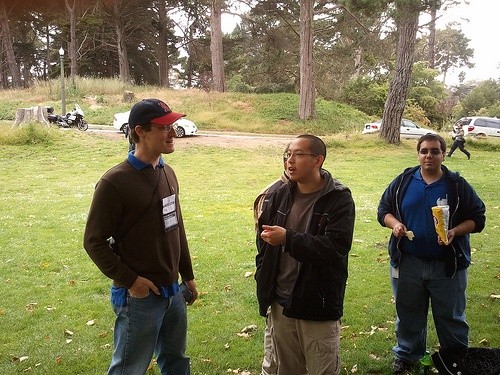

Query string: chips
[406,231,414,240]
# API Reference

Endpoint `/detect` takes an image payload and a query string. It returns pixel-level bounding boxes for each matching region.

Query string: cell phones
[180,282,193,303]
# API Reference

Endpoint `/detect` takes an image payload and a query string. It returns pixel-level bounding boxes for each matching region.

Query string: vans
[452,116,500,139]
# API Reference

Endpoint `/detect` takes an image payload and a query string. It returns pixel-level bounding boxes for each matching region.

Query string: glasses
[147,124,178,133]
[418,147,441,155]
[283,151,318,160]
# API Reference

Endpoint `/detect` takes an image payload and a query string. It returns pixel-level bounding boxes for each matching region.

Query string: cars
[114,109,198,138]
[363,117,437,139]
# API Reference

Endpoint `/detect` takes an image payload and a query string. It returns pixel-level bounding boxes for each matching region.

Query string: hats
[128,98,187,129]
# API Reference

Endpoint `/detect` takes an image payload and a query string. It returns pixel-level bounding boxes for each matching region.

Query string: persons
[254,134,355,375]
[253,140,293,375]
[445,123,471,160]
[377,133,486,374]
[83,99,199,375]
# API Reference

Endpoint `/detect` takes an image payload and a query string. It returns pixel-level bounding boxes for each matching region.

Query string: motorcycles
[45,103,88,131]
